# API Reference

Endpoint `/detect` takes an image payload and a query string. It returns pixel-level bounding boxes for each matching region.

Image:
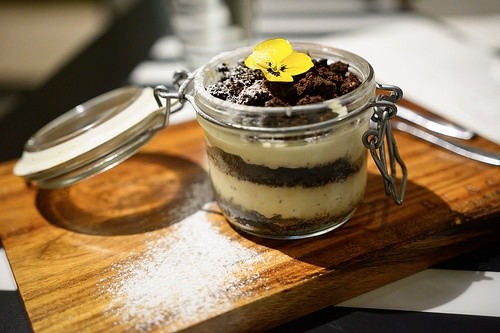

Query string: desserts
[205,54,372,240]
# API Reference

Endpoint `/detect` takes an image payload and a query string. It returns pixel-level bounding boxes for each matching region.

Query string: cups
[189,41,374,239]
